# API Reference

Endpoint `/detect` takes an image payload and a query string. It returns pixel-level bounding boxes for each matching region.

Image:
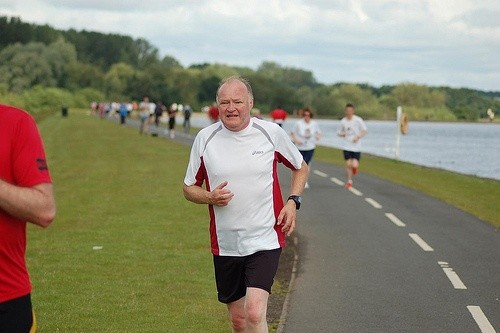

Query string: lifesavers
[401,113,410,134]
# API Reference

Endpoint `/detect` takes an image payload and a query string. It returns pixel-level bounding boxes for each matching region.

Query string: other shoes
[346,182,352,189]
[304,182,309,189]
[352,168,358,176]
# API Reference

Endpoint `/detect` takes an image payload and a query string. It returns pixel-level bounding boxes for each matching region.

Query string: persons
[183,75,308,332]
[336,103,368,189]
[289,106,322,189]
[61,96,305,138]
[0,102,58,333]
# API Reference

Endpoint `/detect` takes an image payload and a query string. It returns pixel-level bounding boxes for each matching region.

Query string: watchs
[286,194,302,210]
